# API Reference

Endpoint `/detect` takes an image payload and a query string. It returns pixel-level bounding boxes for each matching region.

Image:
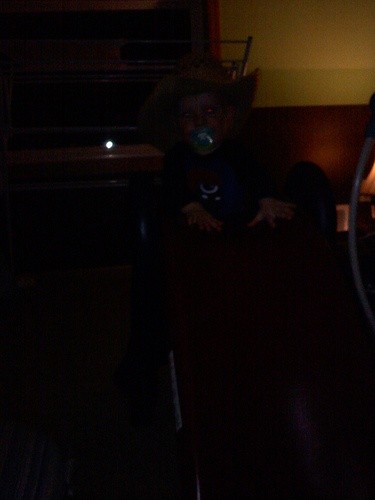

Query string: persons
[109,53,337,429]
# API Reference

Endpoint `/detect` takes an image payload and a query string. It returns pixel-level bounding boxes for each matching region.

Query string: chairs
[114,161,336,422]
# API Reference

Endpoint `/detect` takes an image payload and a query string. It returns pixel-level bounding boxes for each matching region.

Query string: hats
[136,62,260,140]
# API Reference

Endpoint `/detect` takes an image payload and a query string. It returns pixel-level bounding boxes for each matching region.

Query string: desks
[5,144,163,295]
